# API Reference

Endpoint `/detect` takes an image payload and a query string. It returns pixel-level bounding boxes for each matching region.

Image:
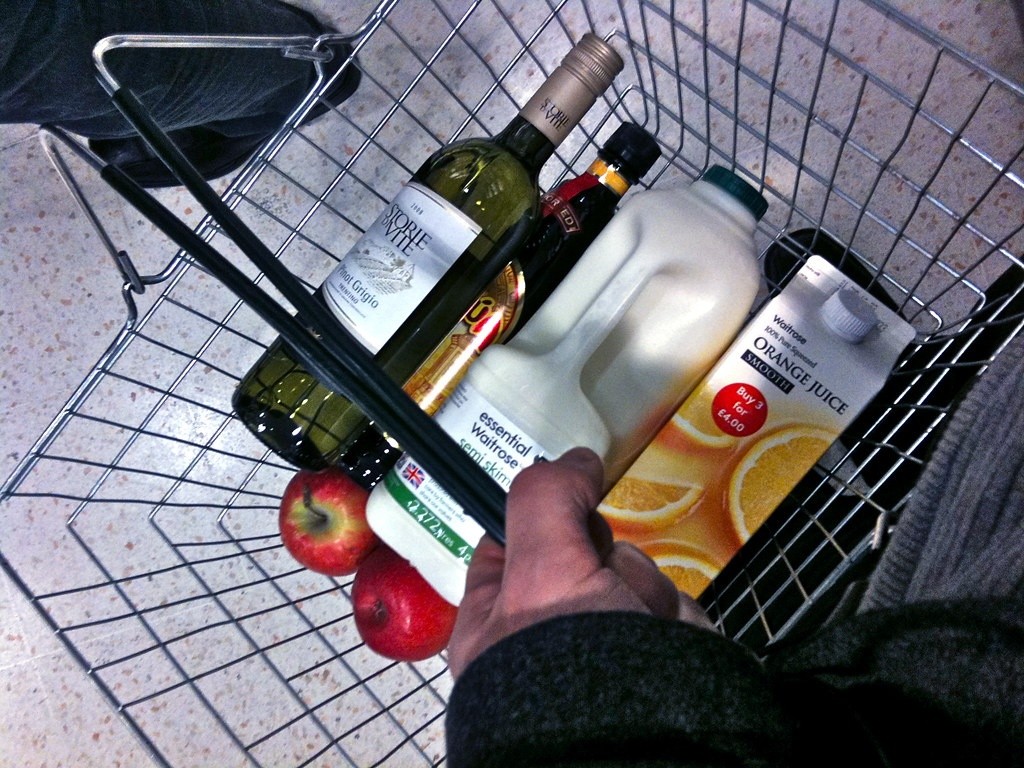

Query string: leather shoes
[86,9,359,187]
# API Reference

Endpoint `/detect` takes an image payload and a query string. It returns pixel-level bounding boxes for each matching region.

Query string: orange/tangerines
[595,384,839,600]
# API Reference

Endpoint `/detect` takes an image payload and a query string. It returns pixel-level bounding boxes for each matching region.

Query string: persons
[1,1,364,189]
[442,222,1024,767]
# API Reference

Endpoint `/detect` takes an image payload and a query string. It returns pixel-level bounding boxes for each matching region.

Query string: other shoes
[763,226,915,485]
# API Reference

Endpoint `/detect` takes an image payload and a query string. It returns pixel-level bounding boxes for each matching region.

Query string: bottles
[230,34,626,476]
[337,121,662,495]
[363,163,770,610]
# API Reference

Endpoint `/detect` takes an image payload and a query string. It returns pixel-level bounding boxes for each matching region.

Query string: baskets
[1,0,1022,768]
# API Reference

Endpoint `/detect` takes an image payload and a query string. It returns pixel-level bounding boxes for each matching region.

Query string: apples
[351,544,457,663]
[277,469,379,577]
